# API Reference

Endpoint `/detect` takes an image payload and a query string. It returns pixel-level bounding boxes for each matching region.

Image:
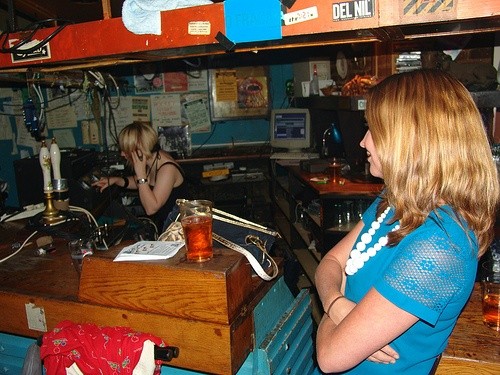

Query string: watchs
[136,179,148,184]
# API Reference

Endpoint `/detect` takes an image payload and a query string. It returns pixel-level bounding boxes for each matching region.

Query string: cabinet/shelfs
[267,158,384,324]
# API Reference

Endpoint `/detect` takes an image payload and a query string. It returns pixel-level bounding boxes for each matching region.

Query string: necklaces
[344,207,400,275]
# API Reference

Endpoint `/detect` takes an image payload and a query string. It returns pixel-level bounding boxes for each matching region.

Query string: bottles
[310,64,319,97]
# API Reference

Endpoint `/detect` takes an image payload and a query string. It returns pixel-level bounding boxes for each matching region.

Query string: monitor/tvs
[270,109,311,152]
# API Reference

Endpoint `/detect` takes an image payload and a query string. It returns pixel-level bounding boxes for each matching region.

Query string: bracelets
[122,177,129,188]
[327,296,345,317]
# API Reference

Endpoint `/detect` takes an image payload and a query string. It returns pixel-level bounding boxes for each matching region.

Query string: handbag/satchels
[161,198,282,280]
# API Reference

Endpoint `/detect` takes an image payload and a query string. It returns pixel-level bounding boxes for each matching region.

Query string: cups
[329,162,341,184]
[333,210,364,230]
[480,274,500,332]
[179,199,214,263]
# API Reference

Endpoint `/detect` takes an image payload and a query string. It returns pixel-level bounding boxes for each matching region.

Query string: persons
[314,70,500,375]
[91,121,186,237]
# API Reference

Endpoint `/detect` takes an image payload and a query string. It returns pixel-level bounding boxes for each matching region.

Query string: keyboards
[270,152,321,159]
